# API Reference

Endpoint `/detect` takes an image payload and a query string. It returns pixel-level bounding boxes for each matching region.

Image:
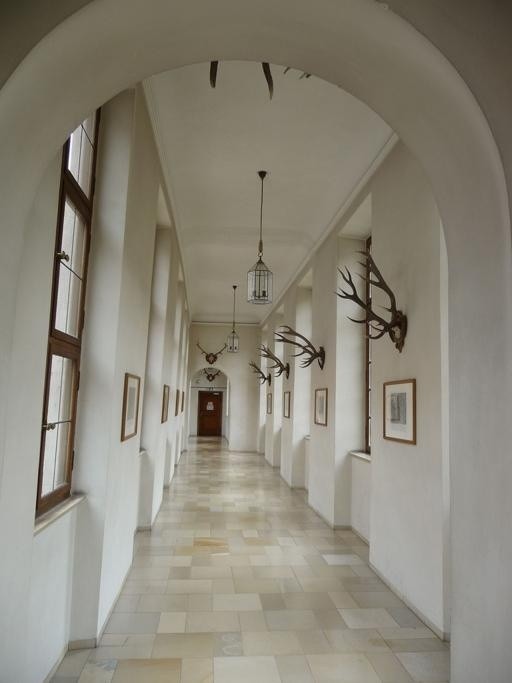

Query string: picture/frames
[120,372,140,443]
[265,387,329,428]
[381,379,418,445]
[160,385,184,422]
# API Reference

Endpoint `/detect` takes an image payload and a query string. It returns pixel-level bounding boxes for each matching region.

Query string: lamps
[246,168,274,307]
[226,286,239,354]
